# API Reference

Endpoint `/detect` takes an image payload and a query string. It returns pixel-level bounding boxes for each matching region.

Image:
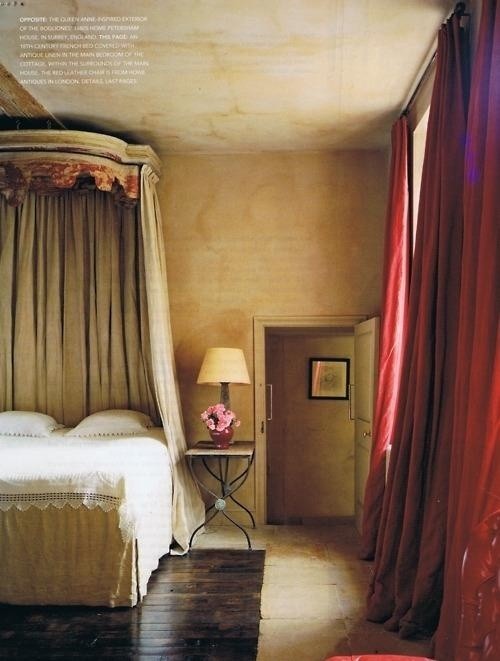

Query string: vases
[208,427,234,449]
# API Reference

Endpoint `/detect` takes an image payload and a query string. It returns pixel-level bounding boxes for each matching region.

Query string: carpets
[0,548,267,661]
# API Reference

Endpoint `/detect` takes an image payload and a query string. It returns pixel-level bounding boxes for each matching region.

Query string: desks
[184,440,257,551]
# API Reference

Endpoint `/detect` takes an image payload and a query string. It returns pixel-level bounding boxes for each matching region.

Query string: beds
[0,427,177,608]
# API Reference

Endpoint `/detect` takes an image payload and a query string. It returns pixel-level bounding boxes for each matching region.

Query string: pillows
[0,410,62,437]
[65,410,154,436]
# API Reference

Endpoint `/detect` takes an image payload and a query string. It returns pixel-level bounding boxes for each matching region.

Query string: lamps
[197,347,251,412]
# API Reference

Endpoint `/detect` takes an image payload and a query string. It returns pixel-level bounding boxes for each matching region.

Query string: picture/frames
[308,357,351,400]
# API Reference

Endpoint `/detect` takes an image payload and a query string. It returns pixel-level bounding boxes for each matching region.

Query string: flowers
[200,404,241,432]
